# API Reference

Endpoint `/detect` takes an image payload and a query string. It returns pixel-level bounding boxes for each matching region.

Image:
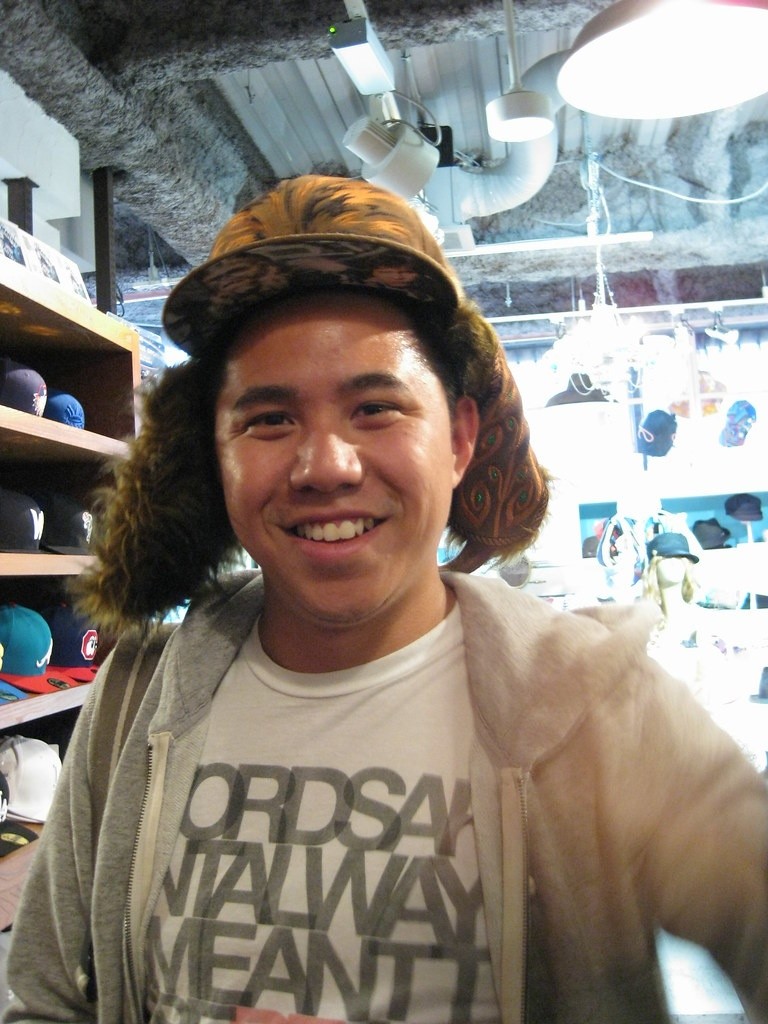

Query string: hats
[723,493,762,519]
[42,383,84,429]
[41,492,93,556]
[0,734,63,858]
[38,603,101,682]
[0,488,44,553]
[160,176,463,357]
[694,518,731,548]
[0,359,47,417]
[718,401,756,448]
[0,600,78,693]
[637,410,677,458]
[646,533,699,562]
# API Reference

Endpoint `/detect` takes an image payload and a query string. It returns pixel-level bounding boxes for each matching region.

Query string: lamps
[557,0,768,122]
[339,89,442,206]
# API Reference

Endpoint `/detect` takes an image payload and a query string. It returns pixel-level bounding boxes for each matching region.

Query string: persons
[640,532,701,612]
[1,175,768,1024]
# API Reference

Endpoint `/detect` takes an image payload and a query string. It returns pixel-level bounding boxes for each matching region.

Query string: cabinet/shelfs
[0,253,139,933]
